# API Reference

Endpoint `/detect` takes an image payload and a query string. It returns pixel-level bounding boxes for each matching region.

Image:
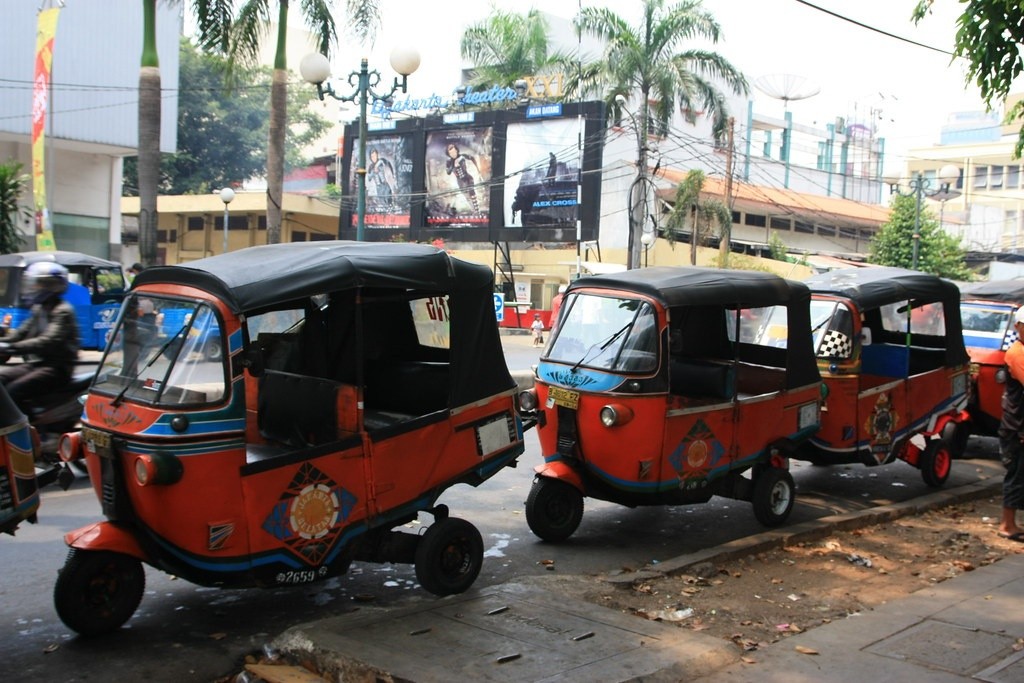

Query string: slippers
[997,532,1024,543]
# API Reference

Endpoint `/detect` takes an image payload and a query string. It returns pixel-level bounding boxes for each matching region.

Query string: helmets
[18,262,69,307]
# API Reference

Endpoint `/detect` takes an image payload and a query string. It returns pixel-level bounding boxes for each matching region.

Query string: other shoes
[532,345,537,348]
[536,345,540,347]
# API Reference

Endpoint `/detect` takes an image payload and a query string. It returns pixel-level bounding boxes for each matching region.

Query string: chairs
[857,344,910,387]
[243,368,362,462]
[668,359,733,411]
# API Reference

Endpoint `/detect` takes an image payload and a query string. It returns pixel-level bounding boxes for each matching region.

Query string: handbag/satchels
[540,336,544,343]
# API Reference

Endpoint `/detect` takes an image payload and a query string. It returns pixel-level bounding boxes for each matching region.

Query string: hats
[133,262,144,271]
[1015,306,1024,323]
[558,285,566,293]
[534,313,540,317]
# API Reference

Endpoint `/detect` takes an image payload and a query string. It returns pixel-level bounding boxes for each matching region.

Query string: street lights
[300,45,422,242]
[616,93,649,270]
[881,163,960,274]
[219,187,236,254]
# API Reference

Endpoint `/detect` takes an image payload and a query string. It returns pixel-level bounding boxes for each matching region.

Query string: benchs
[342,357,451,431]
[889,343,945,378]
[726,359,787,400]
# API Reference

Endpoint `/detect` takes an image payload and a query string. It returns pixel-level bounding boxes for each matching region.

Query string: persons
[122,262,144,290]
[997,305,1024,536]
[545,152,557,188]
[731,309,758,342]
[368,149,395,210]
[75,264,105,295]
[549,285,608,347]
[0,261,79,458]
[445,143,481,212]
[531,312,544,347]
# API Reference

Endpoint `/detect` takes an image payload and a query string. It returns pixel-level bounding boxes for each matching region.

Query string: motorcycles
[0,248,305,537]
[515,263,829,547]
[906,269,1024,442]
[52,240,527,642]
[732,265,973,489]
